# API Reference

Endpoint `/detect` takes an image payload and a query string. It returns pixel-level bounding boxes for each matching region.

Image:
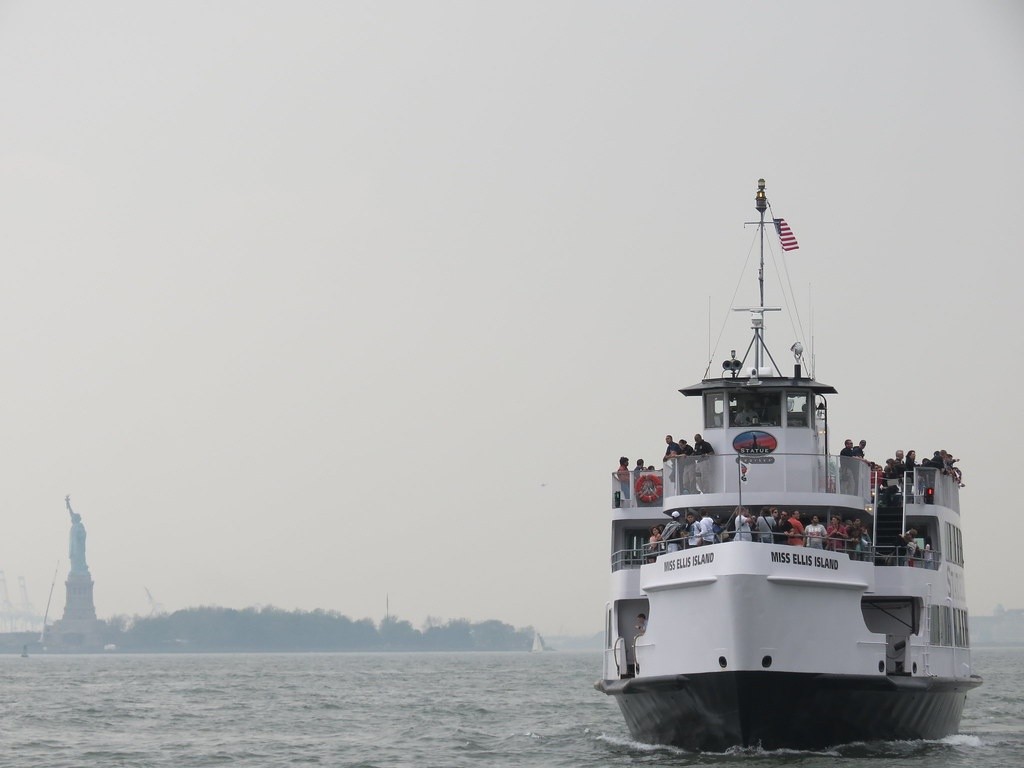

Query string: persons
[892,528,935,570]
[647,508,725,563]
[839,438,866,496]
[869,449,964,506]
[617,457,631,499]
[634,459,655,480]
[661,434,716,494]
[633,613,649,633]
[735,402,760,427]
[727,505,871,562]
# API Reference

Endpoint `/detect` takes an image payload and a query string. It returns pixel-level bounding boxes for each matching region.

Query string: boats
[591,169,982,761]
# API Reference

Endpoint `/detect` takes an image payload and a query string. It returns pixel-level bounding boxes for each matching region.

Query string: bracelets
[638,626,641,629]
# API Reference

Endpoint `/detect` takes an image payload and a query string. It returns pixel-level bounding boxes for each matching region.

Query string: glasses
[781,514,786,517]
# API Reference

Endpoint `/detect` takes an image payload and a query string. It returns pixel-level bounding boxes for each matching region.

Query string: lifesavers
[636,473,662,501]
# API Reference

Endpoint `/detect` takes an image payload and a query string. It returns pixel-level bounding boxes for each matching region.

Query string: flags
[774,218,800,250]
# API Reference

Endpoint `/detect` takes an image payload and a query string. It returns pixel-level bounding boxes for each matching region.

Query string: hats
[671,511,680,518]
[860,440,866,446]
